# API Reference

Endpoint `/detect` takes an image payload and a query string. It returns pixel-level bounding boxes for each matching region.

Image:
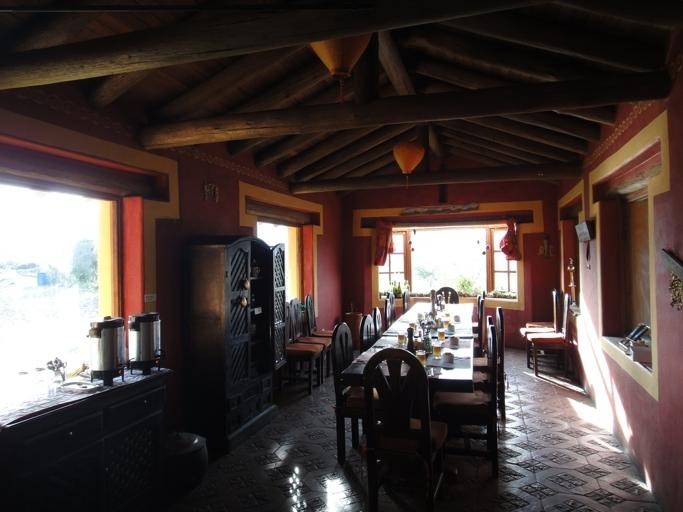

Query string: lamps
[392,139,425,190]
[309,32,372,104]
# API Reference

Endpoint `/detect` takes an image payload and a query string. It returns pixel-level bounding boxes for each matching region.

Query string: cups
[432,317,450,359]
[416,349,427,367]
[408,322,416,333]
[398,331,406,345]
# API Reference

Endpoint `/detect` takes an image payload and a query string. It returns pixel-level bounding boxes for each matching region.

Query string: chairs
[330,286,505,511]
[285,295,333,396]
[517,289,574,378]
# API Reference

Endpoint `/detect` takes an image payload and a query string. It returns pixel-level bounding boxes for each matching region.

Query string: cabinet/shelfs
[0,369,170,510]
[182,235,287,451]
